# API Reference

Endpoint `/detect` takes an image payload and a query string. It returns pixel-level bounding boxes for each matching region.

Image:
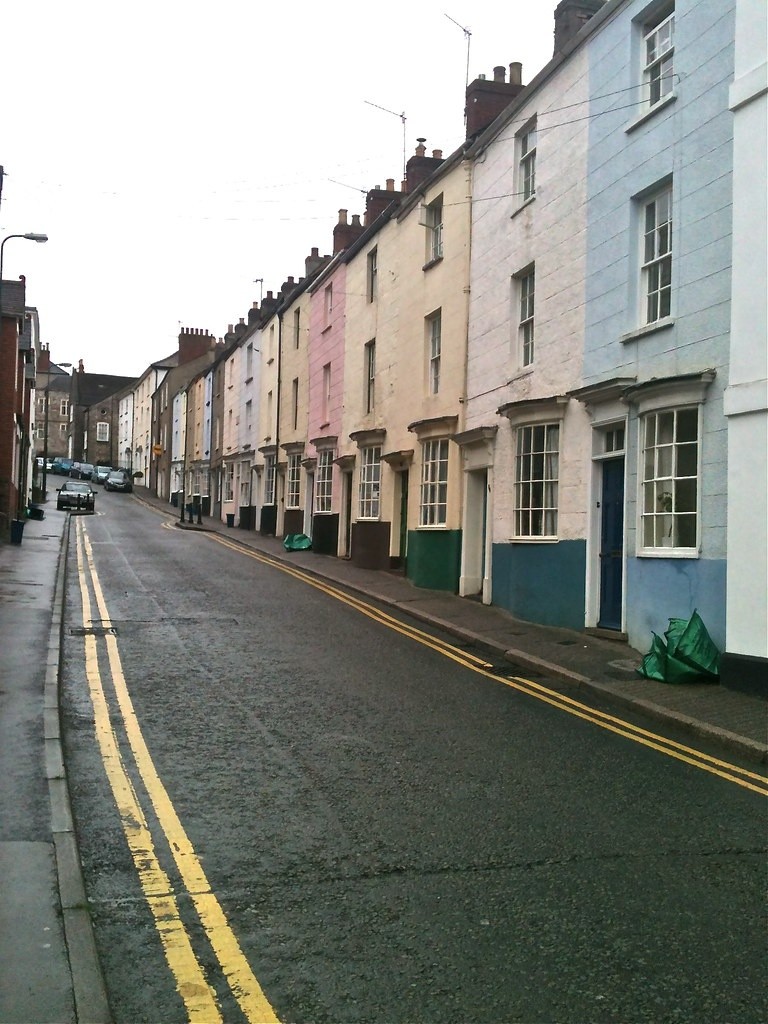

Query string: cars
[104,471,132,493]
[90,466,113,484]
[36,457,53,473]
[70,463,94,480]
[56,481,98,511]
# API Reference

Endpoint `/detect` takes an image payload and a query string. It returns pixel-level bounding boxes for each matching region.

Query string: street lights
[43,363,72,501]
[0,233,48,326]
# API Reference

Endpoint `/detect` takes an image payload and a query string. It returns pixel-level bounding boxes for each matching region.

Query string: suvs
[52,457,74,476]
